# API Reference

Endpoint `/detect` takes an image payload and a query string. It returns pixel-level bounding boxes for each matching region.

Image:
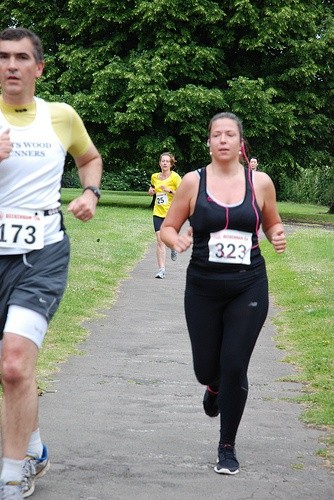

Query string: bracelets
[169,190,173,193]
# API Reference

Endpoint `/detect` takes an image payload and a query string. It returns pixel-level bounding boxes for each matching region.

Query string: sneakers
[171,249,178,261]
[19,445,50,498]
[214,442,240,475]
[203,386,223,417]
[155,269,166,279]
[0,480,23,500]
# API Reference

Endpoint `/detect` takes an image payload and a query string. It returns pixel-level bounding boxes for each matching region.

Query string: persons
[0,28,103,500]
[148,152,183,278]
[249,158,258,171]
[160,112,287,474]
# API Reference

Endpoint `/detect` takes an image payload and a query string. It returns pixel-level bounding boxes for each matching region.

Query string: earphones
[206,141,209,147]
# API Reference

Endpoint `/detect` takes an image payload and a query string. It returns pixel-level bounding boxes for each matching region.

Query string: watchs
[82,186,102,199]
